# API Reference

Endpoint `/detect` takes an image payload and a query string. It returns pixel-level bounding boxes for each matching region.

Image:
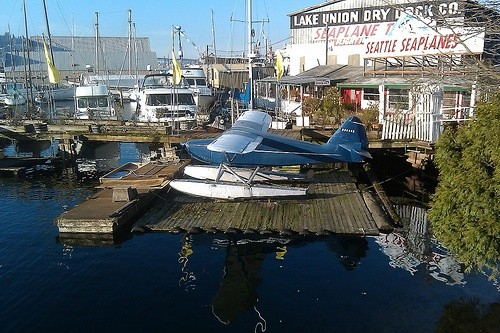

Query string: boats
[1,0,374,201]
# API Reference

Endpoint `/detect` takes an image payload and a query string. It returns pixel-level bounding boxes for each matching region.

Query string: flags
[43,43,60,83]
[172,53,181,85]
[277,49,285,82]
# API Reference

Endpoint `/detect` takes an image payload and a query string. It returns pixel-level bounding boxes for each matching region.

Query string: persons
[285,120,293,129]
[203,95,231,129]
